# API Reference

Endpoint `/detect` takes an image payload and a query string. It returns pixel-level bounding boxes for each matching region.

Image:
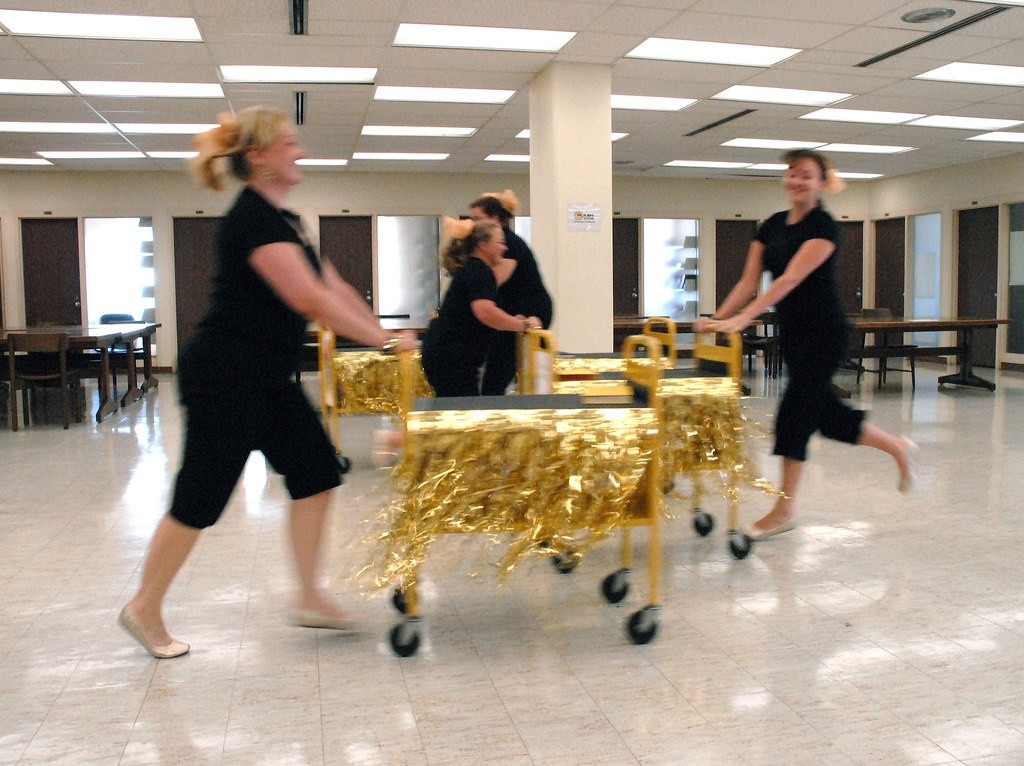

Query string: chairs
[96,313,143,387]
[7,333,82,430]
[856,308,919,391]
[737,307,781,380]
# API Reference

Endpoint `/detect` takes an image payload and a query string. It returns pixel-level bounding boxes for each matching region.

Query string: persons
[431,187,552,398]
[370,221,539,468]
[695,149,918,542]
[120,105,412,658]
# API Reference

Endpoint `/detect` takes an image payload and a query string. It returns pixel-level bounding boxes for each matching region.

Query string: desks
[700,312,862,380]
[614,320,763,395]
[831,318,1012,399]
[0,323,162,424]
[296,317,431,413]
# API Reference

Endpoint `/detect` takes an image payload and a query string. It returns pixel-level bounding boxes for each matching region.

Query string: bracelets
[525,319,529,331]
[383,336,399,352]
[710,316,721,320]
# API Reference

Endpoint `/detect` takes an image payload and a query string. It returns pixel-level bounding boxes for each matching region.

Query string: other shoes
[741,517,796,540]
[898,436,920,494]
[289,605,366,631]
[117,605,190,659]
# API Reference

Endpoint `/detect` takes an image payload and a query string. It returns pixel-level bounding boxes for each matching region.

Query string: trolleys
[518,319,753,560]
[375,334,665,659]
[314,325,432,473]
[511,314,678,495]
